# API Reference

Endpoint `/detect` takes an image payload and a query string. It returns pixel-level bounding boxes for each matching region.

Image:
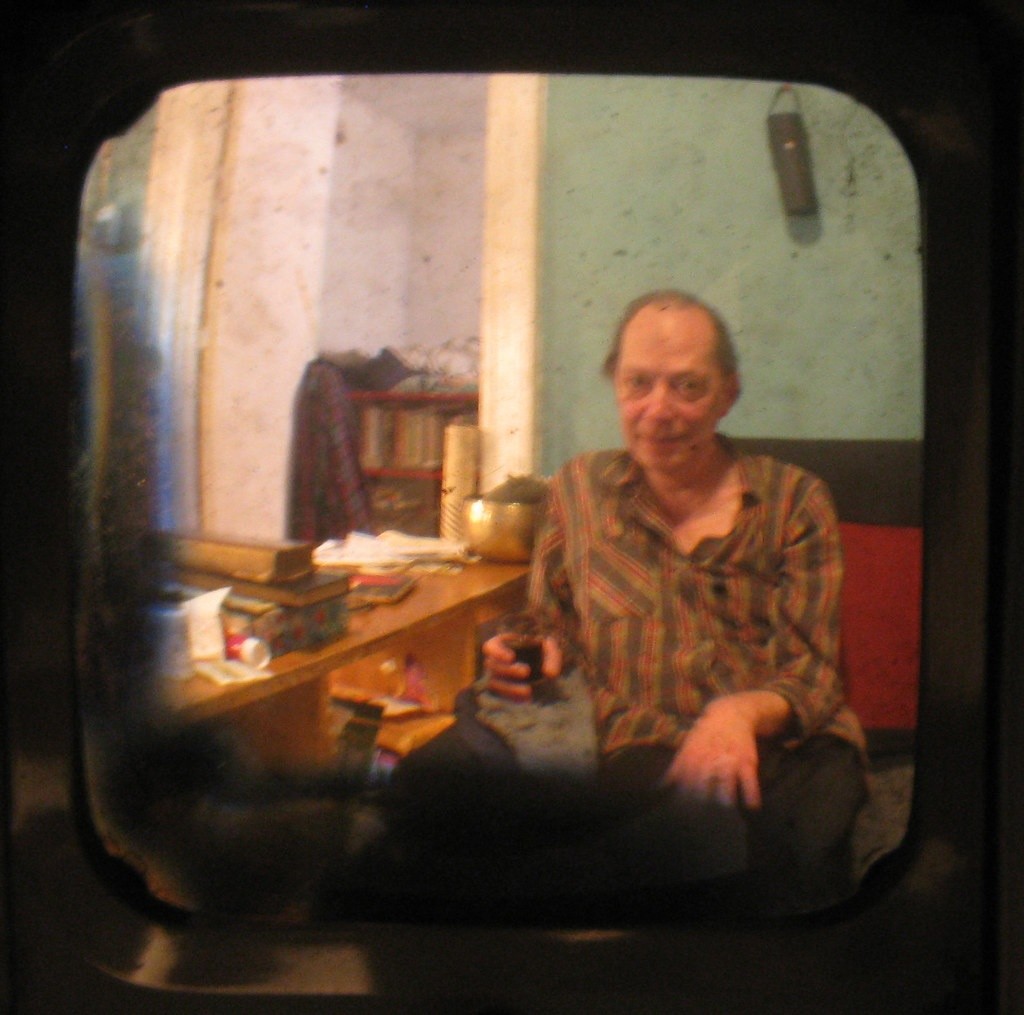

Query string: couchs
[391,436,923,905]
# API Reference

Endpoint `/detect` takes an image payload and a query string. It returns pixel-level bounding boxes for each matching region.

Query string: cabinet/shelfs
[339,388,480,537]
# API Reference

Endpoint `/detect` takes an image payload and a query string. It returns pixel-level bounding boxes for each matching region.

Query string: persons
[483,288,869,908]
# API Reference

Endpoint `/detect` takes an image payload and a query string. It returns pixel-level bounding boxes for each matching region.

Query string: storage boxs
[219,596,346,659]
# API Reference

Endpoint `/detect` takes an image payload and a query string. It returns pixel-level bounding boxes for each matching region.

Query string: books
[354,406,447,470]
[177,568,352,607]
[171,531,318,585]
[356,572,421,604]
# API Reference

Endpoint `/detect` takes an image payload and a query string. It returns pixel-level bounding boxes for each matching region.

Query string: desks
[164,554,528,773]
